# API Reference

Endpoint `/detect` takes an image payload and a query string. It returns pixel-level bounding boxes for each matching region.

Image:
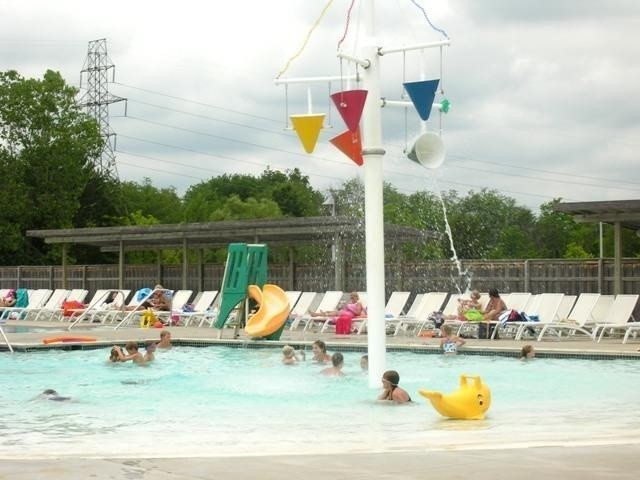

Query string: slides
[245,284,290,338]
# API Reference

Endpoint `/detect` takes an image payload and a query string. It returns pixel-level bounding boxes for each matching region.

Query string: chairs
[489,293,640,344]
[281,291,490,338]
[1,289,236,330]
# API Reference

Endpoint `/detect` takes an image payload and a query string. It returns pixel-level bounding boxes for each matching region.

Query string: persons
[281,340,369,377]
[376,370,413,403]
[0,292,18,307]
[308,292,362,318]
[100,283,173,366]
[458,289,507,322]
[438,323,466,357]
[520,344,534,361]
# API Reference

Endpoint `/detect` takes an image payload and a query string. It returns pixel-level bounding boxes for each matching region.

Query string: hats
[383,371,399,384]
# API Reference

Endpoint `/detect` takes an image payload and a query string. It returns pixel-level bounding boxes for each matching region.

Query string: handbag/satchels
[464,310,482,321]
[183,304,192,312]
[64,302,81,316]
[336,316,351,334]
